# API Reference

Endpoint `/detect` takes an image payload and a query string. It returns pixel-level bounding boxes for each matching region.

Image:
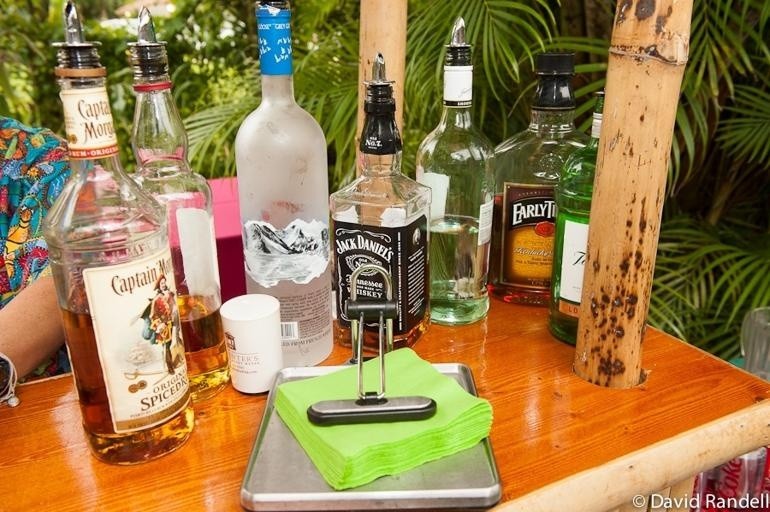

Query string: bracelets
[0,353,19,407]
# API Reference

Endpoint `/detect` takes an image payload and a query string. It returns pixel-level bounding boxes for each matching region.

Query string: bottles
[547,91,606,347]
[125,40,231,402]
[234,7,335,366]
[487,72,590,308]
[42,40,196,465]
[415,46,498,327]
[329,84,432,358]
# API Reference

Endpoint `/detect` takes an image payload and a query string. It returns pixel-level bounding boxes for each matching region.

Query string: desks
[0,283,770,511]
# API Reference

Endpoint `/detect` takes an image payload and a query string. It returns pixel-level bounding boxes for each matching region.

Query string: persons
[1,114,66,409]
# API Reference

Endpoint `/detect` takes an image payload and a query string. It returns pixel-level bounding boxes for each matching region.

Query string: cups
[219,293,284,395]
[741,306,770,382]
